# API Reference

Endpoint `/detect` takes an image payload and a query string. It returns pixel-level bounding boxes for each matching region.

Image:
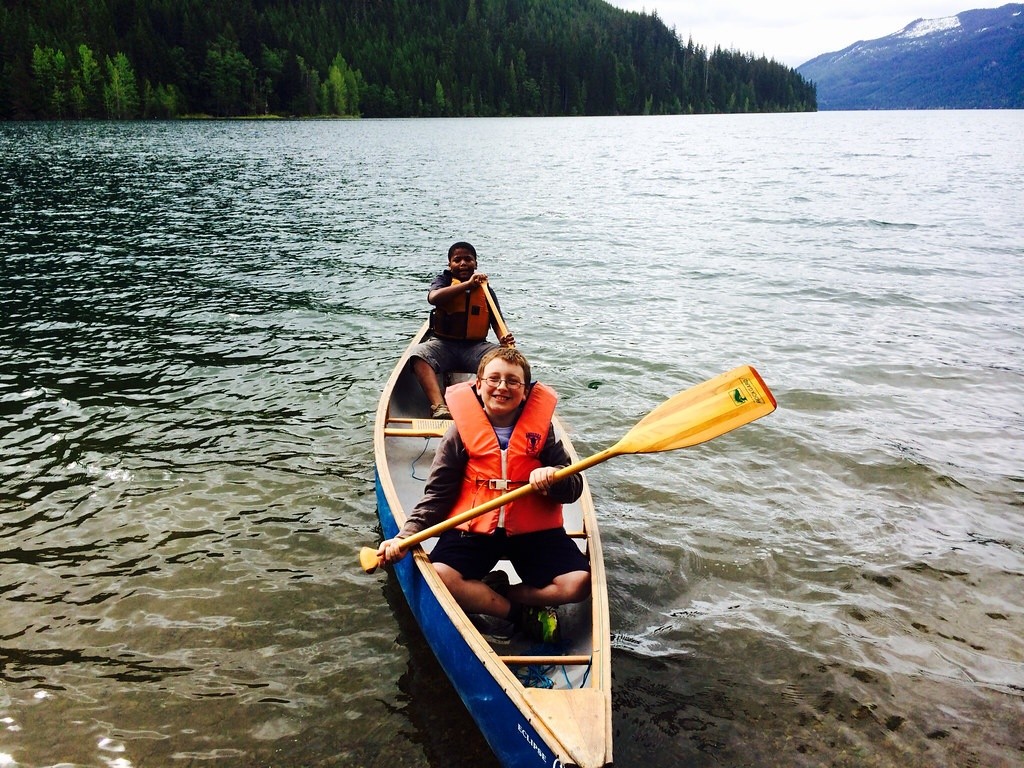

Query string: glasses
[480,377,527,390]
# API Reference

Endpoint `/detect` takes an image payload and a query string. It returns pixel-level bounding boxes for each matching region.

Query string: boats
[375,312,615,768]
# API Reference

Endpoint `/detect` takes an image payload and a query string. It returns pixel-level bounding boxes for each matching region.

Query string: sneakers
[529,606,560,645]
[430,404,450,419]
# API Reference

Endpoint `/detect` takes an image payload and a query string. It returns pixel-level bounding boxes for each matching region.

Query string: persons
[409,242,515,419]
[377,349,591,655]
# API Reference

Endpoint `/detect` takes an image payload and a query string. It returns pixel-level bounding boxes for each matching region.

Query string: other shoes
[481,569,509,589]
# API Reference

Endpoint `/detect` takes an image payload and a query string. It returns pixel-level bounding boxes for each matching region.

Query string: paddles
[473,270,518,351]
[361,366,777,573]
